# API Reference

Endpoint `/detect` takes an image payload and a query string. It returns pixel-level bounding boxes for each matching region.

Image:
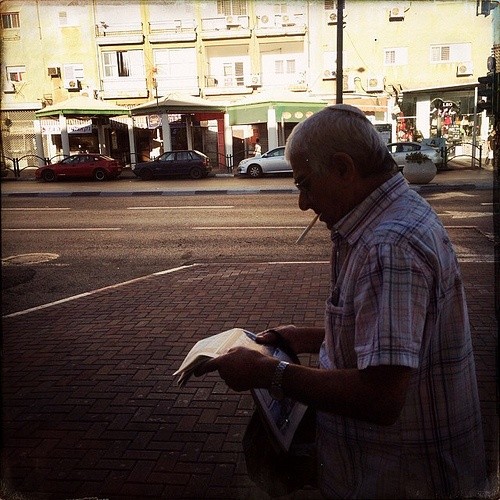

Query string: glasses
[295,167,324,193]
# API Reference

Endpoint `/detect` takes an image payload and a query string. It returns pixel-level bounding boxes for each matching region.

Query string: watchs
[268,361,290,401]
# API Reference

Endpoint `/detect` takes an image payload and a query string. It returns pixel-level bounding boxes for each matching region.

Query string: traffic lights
[477,74,496,116]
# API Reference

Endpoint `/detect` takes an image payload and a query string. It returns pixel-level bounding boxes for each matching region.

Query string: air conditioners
[65,79,79,89]
[259,14,275,27]
[343,74,356,92]
[457,63,474,76]
[323,68,337,79]
[327,10,337,24]
[368,78,385,91]
[225,16,239,26]
[47,67,60,77]
[390,5,404,18]
[249,72,262,86]
[282,13,296,26]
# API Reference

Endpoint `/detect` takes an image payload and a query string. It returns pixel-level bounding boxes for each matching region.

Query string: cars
[35,153,124,183]
[237,146,294,178]
[384,141,444,174]
[133,149,212,180]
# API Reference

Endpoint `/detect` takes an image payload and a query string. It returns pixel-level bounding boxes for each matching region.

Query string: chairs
[396,145,404,152]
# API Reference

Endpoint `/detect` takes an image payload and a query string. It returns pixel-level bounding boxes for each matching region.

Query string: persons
[192,106,485,499]
